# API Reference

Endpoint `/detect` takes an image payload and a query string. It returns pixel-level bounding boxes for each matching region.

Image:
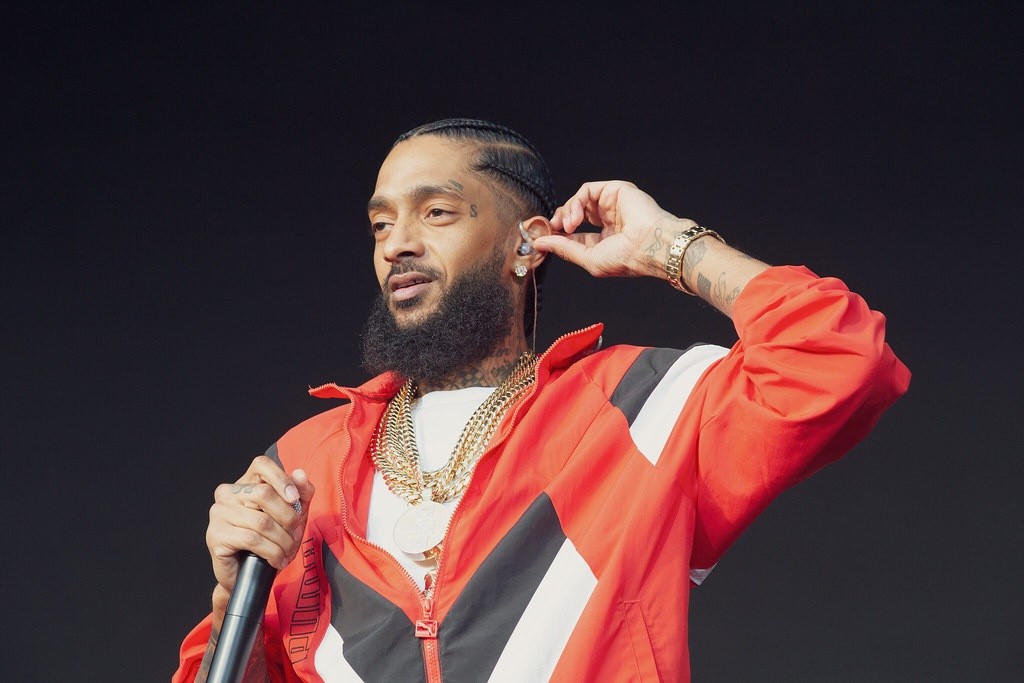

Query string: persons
[168,113,912,682]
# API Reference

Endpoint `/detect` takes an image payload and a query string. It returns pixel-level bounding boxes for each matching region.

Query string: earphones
[517,223,538,256]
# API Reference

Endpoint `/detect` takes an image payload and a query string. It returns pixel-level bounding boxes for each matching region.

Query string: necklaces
[370,352,547,562]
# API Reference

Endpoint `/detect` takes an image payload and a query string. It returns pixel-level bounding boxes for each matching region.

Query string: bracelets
[665,226,724,297]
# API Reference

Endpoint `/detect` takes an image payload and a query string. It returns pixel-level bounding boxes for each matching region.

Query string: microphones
[204,497,303,683]
